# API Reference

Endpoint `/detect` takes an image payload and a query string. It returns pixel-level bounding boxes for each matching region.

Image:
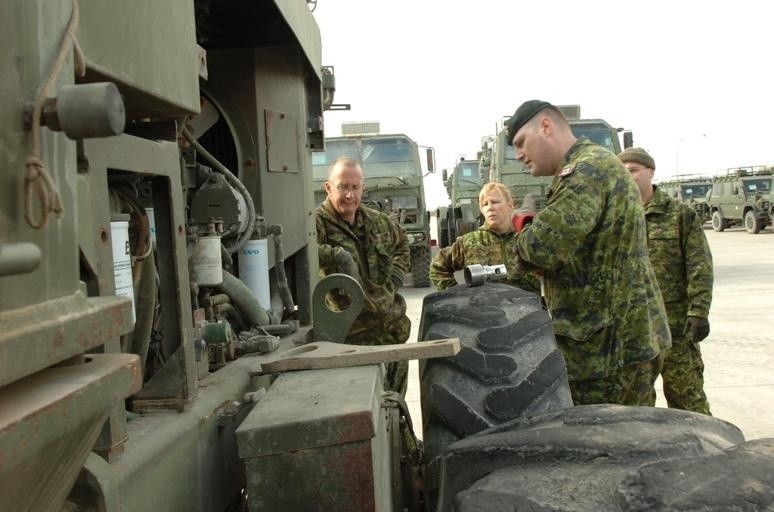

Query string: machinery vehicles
[0,2,773,510]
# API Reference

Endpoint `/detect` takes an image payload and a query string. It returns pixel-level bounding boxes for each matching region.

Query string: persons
[507,99,674,408]
[313,156,411,398]
[615,147,713,417]
[428,182,542,304]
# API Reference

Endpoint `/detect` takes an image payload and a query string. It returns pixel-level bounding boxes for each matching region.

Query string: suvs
[651,174,714,230]
[704,166,773,234]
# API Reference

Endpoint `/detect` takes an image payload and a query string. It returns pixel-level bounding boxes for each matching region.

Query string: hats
[616,147,655,169]
[506,101,550,145]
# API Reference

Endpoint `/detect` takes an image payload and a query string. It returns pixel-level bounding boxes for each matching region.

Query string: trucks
[452,104,635,248]
[309,122,435,291]
[436,146,485,250]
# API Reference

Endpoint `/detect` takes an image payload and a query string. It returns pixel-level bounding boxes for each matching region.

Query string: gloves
[334,247,359,296]
[510,193,536,230]
[682,317,710,343]
